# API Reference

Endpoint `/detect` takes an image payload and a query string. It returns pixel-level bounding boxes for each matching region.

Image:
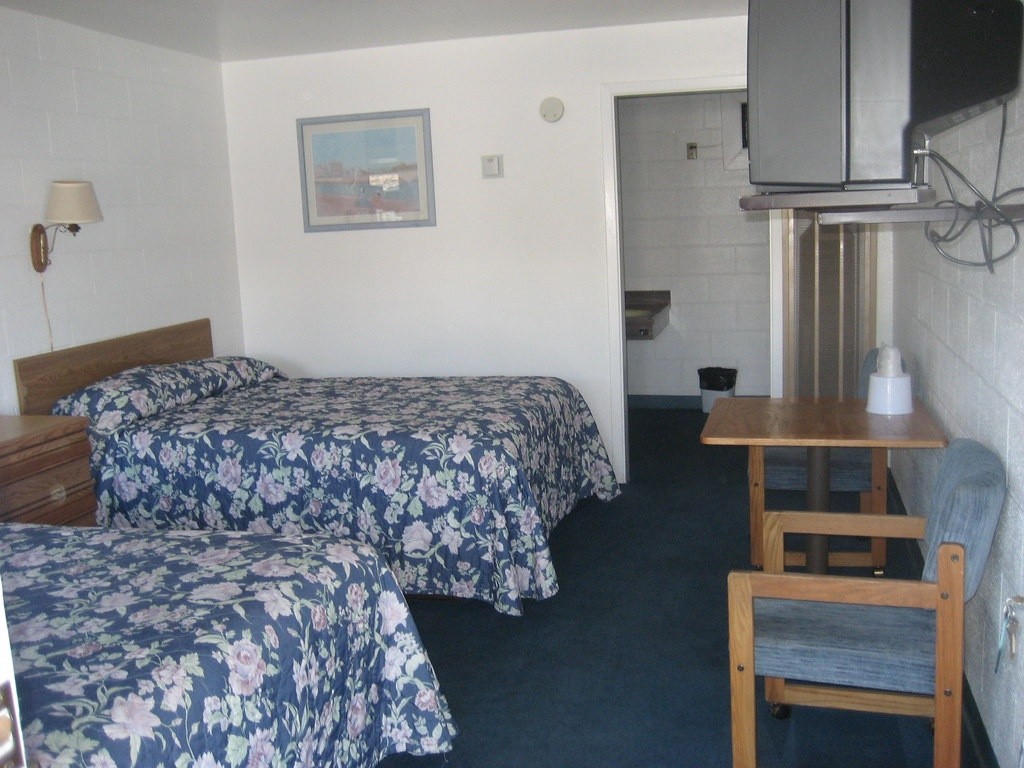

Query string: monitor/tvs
[745,0,1021,195]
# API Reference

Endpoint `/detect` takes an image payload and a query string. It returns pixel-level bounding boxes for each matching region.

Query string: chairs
[728,438,1007,768]
[746,348,906,543]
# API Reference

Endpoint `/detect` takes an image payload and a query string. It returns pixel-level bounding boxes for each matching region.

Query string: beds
[14,318,621,616]
[1,523,460,768]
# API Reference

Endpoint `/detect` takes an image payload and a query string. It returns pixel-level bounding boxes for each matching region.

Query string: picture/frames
[297,108,436,232]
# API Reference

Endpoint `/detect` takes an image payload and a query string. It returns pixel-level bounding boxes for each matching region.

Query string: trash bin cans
[697,366,738,414]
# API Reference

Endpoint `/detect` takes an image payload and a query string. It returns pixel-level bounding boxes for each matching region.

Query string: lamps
[31,182,104,273]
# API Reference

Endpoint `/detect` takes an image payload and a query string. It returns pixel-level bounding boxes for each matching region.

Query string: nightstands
[0,415,97,528]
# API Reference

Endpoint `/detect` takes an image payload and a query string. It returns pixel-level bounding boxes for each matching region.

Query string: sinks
[625,307,671,341]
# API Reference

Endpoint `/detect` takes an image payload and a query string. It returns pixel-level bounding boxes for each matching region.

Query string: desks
[701,398,949,574]
